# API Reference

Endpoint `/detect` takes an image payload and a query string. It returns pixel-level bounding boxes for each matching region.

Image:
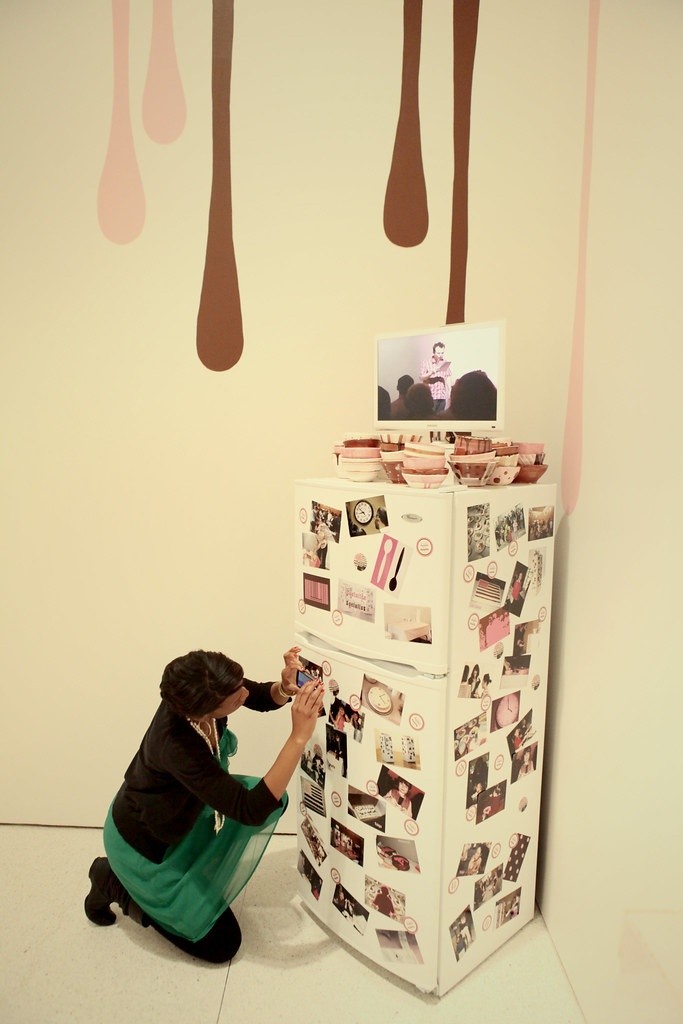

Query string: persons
[468,758,501,802]
[85,647,325,964]
[327,707,362,758]
[495,509,553,549]
[378,343,497,421]
[297,821,410,923]
[312,504,342,568]
[383,777,412,818]
[302,661,321,689]
[302,745,324,784]
[458,664,492,698]
[480,572,528,673]
[510,720,536,784]
[458,844,489,876]
[351,507,387,537]
[453,870,520,955]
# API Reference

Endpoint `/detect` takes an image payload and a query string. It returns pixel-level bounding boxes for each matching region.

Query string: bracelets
[278,684,296,697]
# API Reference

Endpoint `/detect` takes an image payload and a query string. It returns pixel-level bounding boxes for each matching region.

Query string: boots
[85,857,150,927]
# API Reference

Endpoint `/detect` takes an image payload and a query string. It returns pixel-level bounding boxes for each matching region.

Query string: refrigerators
[295,475,555,998]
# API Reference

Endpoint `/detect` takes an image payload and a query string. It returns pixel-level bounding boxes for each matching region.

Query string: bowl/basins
[331,433,548,489]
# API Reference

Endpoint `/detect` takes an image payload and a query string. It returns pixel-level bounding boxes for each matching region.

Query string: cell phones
[296,669,317,692]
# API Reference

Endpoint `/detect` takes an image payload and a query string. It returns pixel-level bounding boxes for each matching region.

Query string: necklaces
[188,718,225,835]
